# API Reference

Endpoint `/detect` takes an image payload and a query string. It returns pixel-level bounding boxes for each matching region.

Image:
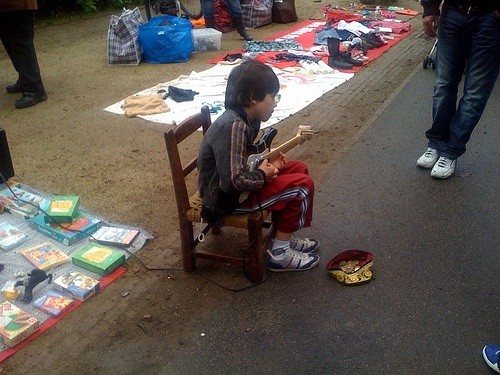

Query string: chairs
[164,106,274,281]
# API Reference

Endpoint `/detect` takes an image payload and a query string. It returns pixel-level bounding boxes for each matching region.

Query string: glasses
[275,94,281,103]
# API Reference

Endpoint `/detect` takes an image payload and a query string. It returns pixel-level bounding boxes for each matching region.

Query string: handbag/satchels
[106,7,144,66]
[138,15,193,64]
[240,0,273,29]
[272,0,297,23]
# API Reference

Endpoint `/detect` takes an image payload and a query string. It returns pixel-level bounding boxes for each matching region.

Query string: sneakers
[271,234,320,253]
[16,91,47,108]
[482,344,500,374]
[416,147,439,168]
[431,156,457,179]
[6,83,31,93]
[266,248,320,271]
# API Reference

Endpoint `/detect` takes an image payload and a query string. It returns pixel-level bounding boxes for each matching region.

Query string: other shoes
[348,32,388,62]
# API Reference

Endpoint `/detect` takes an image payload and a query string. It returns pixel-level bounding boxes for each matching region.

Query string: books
[0,222,29,251]
[21,241,70,271]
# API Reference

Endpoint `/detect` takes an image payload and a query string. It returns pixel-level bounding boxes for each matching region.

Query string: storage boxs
[0,184,126,348]
[192,28,223,52]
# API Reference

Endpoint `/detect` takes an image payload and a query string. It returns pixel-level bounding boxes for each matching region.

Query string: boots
[340,51,363,66]
[327,37,353,69]
[233,16,253,41]
[205,17,215,29]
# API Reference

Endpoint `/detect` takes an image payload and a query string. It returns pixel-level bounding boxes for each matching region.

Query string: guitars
[244,124,314,173]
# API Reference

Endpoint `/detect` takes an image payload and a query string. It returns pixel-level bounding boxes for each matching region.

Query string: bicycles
[145,0,203,21]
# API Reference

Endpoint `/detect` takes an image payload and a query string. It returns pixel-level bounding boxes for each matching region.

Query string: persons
[197,59,320,271]
[202,0,252,40]
[417,0,500,178]
[0,0,48,108]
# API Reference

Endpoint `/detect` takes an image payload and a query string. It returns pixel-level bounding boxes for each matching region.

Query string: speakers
[0,128,15,184]
